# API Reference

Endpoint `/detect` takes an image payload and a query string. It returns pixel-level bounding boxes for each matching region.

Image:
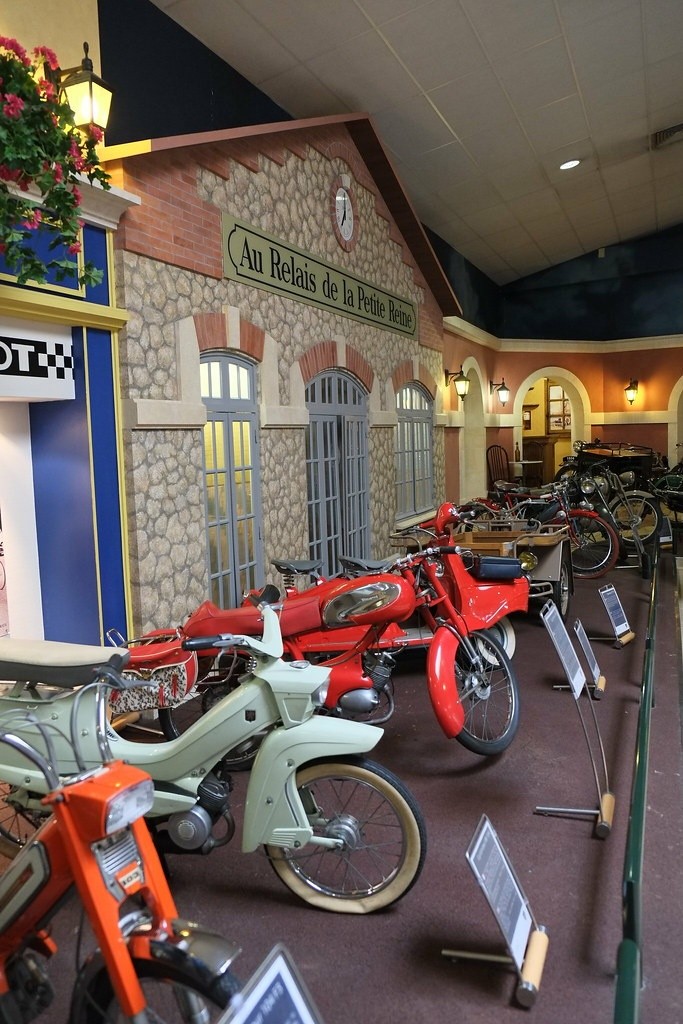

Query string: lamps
[490,377,510,407]
[624,377,639,405]
[44,42,114,140]
[445,365,470,402]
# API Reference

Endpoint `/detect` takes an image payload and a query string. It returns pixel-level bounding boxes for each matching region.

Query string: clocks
[336,187,353,240]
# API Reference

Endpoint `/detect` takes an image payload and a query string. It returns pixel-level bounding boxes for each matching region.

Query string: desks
[505,461,542,487]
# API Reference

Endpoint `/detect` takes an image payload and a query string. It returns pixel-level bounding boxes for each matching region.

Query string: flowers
[0,36,112,287]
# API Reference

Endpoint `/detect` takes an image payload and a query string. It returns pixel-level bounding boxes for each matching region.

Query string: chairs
[521,441,543,487]
[487,445,518,487]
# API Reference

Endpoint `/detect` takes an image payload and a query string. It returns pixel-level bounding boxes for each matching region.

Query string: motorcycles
[0,592,427,914]
[328,439,683,671]
[144,502,530,755]
[0,655,324,1024]
